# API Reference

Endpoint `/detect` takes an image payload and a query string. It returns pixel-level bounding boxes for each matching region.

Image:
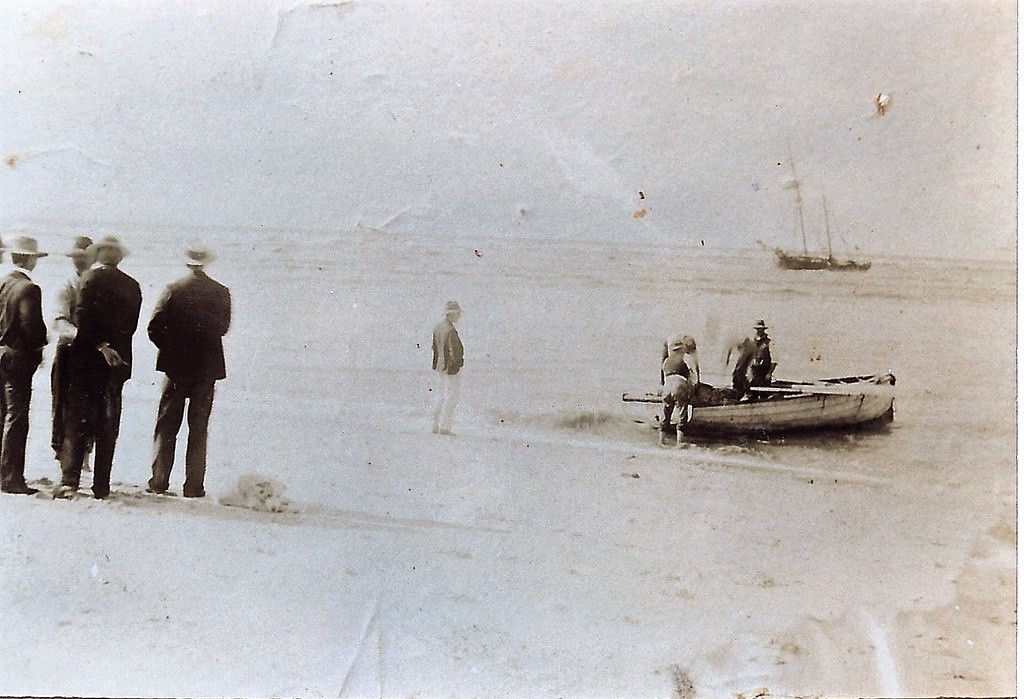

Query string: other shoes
[183,488,205,498]
[440,428,456,436]
[656,429,671,449]
[0,480,39,494]
[676,430,689,449]
[54,485,78,500]
[146,483,166,494]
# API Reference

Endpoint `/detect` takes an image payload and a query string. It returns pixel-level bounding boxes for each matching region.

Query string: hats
[181,245,217,266]
[66,237,93,255]
[7,235,48,257]
[754,320,768,329]
[683,334,696,353]
[441,301,462,314]
[89,236,130,259]
[669,340,684,350]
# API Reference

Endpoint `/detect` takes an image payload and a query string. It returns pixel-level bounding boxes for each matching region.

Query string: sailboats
[776,133,873,272]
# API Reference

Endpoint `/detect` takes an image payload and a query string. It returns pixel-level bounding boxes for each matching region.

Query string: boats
[622,371,896,432]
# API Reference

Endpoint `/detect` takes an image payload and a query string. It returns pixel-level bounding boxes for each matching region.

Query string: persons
[431,300,465,437]
[1,237,233,498]
[658,319,774,448]
